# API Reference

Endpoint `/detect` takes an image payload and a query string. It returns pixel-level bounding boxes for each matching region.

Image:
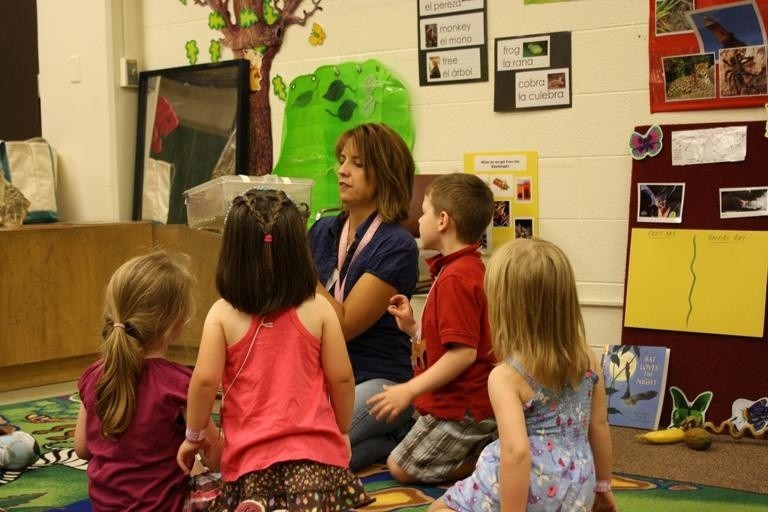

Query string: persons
[306,123,419,471]
[74,249,223,512]
[177,187,376,512]
[427,237,617,512]
[367,173,499,483]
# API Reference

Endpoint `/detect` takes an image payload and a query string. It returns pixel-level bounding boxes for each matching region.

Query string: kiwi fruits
[684,429,714,450]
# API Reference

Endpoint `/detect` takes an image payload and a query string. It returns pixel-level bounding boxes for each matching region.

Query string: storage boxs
[181,175,317,232]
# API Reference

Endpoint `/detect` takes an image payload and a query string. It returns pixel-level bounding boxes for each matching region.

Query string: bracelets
[185,423,208,443]
[596,479,611,493]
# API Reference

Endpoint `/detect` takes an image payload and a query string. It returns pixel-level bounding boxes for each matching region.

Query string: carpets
[1,389,767,510]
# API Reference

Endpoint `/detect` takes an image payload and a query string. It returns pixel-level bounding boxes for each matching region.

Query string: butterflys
[669,388,711,429]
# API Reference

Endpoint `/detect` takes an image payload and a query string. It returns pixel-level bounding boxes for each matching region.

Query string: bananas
[640,429,684,446]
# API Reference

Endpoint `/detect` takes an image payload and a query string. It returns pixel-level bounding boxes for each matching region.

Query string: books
[600,344,671,431]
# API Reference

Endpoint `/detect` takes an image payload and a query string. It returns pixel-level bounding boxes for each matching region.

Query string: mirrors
[132,58,251,225]
